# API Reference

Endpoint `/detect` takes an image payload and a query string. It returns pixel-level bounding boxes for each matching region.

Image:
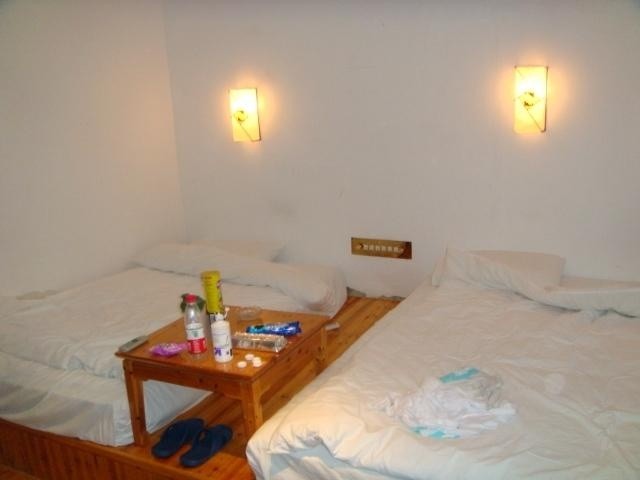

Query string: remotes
[118,335,148,353]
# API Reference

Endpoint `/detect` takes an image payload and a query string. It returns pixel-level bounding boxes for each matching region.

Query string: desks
[115,305,333,448]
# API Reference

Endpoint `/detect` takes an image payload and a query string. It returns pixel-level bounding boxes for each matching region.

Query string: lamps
[513,64,550,130]
[228,88,260,142]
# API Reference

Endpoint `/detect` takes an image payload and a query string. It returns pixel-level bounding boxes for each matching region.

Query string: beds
[0,242,348,447]
[246,249,639,480]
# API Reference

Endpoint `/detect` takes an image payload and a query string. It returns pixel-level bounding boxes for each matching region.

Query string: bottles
[183,295,208,361]
[212,313,233,362]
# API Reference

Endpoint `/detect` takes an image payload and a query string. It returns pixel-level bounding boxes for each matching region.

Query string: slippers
[152,418,203,457]
[181,424,233,466]
